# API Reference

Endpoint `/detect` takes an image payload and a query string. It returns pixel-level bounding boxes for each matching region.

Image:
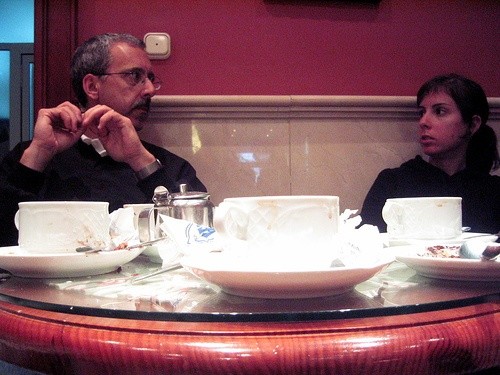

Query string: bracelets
[132,159,162,181]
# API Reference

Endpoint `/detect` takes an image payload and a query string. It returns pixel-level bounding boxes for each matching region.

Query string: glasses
[95,70,163,90]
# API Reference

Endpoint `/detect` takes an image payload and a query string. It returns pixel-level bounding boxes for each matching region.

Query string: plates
[396,243,500,281]
[378,233,498,247]
[181,254,396,298]
[0,246,146,278]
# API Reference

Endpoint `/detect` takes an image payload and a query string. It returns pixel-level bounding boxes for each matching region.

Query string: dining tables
[0,251,500,375]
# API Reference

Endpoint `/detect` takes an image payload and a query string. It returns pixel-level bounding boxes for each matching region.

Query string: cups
[14,202,109,254]
[214,196,339,266]
[382,197,463,238]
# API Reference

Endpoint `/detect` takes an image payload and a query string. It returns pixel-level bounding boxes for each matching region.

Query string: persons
[0,31,207,247]
[356,72,500,244]
